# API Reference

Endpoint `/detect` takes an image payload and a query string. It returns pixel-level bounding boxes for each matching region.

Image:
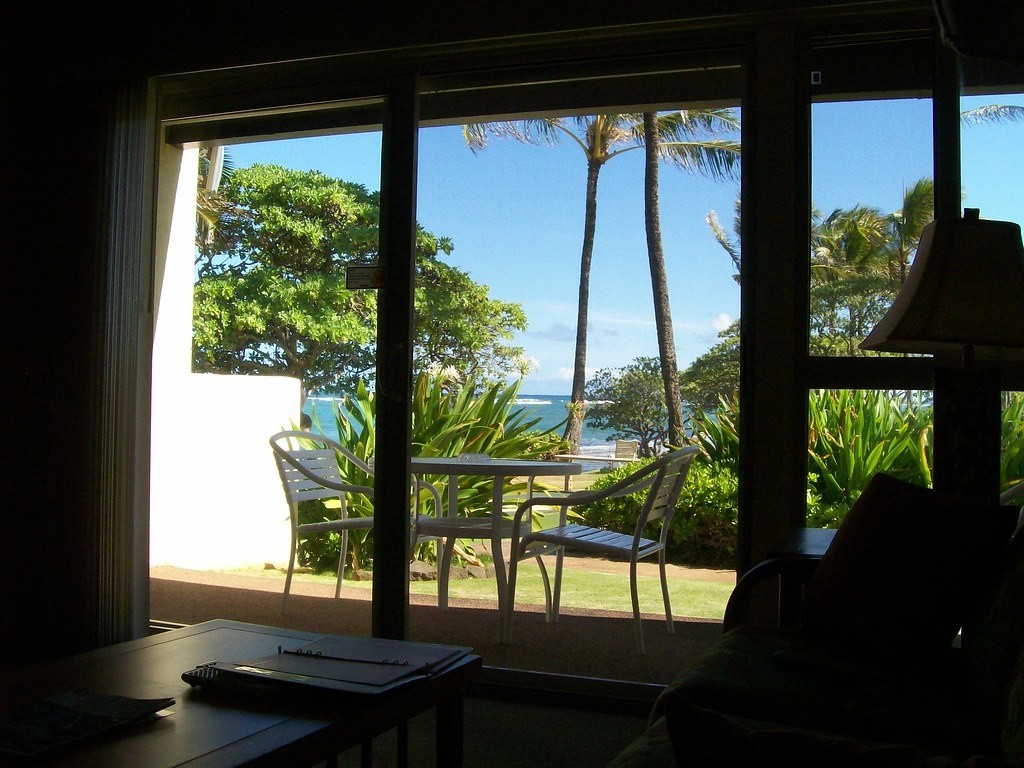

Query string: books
[212,636,473,695]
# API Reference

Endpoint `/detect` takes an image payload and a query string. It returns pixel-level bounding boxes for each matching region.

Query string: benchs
[606,557,1024,768]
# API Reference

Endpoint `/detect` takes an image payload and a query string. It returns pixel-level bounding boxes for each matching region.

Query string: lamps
[858,209,1024,505]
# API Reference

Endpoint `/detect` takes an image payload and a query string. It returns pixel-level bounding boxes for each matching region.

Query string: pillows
[803,472,1020,643]
[663,690,928,768]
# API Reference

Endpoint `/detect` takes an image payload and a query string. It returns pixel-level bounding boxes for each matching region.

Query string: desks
[554,454,640,491]
[366,456,582,643]
[0,619,483,768]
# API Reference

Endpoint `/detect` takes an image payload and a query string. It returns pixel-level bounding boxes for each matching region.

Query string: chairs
[269,430,443,615]
[501,444,701,657]
[615,439,638,458]
